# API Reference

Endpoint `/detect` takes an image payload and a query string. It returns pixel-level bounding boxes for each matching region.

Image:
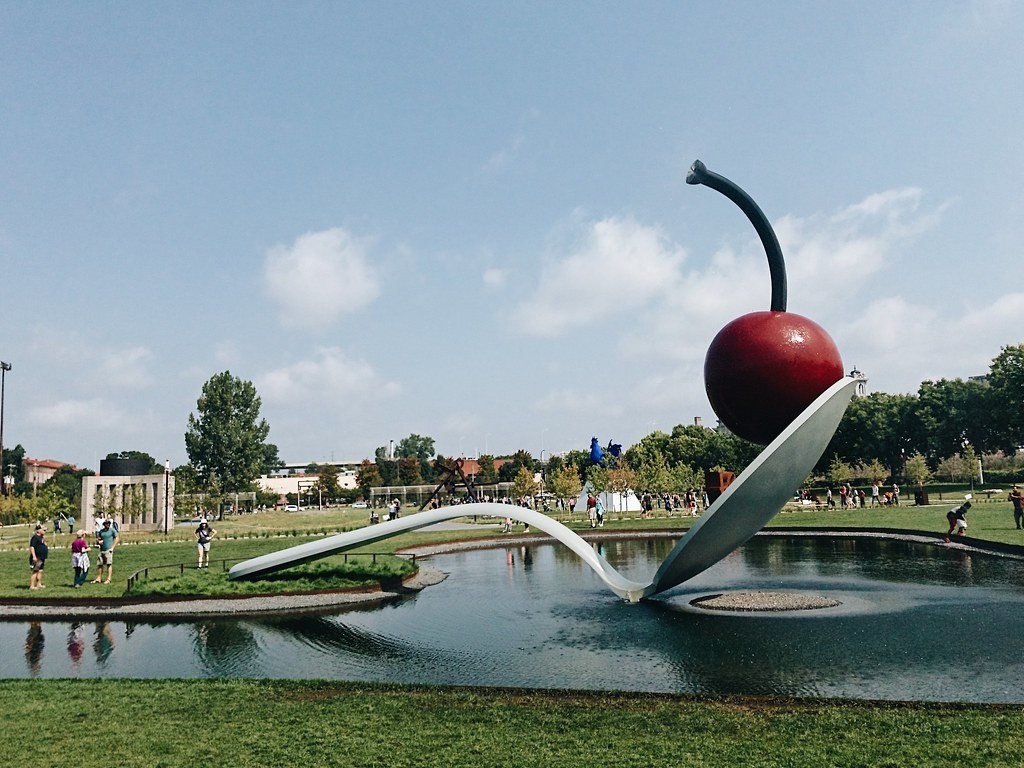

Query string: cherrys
[685,160,844,446]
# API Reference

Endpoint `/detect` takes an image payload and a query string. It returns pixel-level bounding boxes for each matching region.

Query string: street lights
[902,448,910,500]
[165,459,170,535]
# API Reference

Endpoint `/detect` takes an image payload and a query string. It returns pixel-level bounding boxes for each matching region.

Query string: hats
[77,530,88,537]
[103,519,111,525]
[36,524,47,530]
[201,519,207,523]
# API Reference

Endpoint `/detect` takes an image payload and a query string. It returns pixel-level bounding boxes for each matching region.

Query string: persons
[386,486,710,536]
[28,524,48,589]
[53,514,64,535]
[68,515,75,535]
[1008,486,1024,529]
[195,502,277,520]
[193,519,217,570]
[104,513,114,529]
[956,506,968,536]
[90,520,119,584]
[94,512,106,548]
[790,483,903,509]
[71,529,92,588]
[943,501,971,544]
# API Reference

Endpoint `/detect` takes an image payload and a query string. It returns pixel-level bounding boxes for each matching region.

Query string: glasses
[105,522,111,524]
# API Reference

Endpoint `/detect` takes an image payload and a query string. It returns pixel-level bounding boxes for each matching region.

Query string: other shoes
[75,584,81,587]
[942,536,950,542]
[91,580,102,583]
[37,585,46,589]
[957,532,966,536]
[30,587,37,590]
[104,580,111,584]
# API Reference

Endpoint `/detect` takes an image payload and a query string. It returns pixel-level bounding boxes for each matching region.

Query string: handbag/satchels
[957,519,967,527]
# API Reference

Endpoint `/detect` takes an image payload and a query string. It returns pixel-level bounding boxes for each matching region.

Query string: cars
[351,501,368,508]
[284,505,306,512]
